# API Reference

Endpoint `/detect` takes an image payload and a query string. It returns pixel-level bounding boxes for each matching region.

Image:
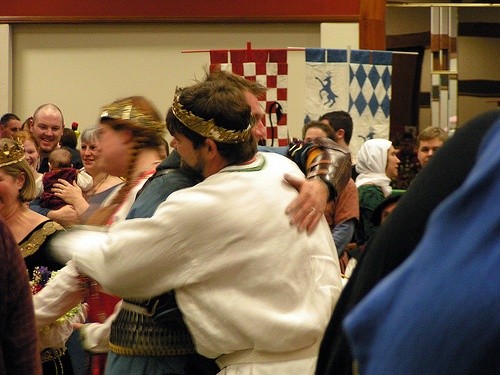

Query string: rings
[313,208,318,212]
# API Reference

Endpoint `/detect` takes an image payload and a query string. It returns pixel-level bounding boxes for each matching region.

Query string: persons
[48,80,343,375]
[342,109,500,375]
[315,109,500,375]
[0,217,44,375]
[0,70,500,375]
[0,138,89,375]
[33,97,169,375]
[106,69,352,375]
